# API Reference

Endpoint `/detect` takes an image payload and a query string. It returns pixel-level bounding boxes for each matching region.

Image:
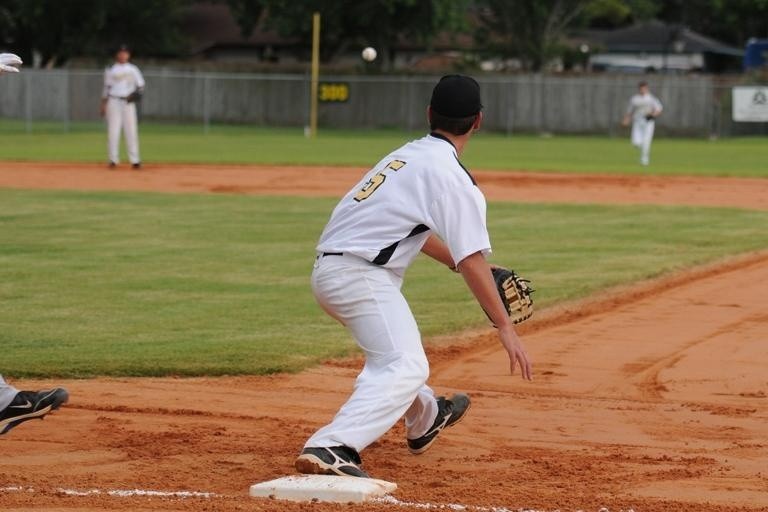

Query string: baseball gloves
[127,87,142,102]
[482,267,533,328]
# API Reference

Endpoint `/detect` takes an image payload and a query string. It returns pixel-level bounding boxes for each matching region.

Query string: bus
[584,52,706,77]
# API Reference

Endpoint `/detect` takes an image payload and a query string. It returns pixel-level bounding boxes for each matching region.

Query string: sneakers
[293,444,372,480]
[407,392,472,456]
[0,388,70,435]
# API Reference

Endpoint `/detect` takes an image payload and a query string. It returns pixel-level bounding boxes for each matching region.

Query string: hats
[429,73,482,120]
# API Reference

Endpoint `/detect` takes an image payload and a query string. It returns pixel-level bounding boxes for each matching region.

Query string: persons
[294,75,533,478]
[622,81,664,165]
[100,44,146,170]
[0,52,70,436]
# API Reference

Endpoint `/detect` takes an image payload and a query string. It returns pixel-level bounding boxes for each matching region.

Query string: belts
[315,251,344,260]
[107,93,128,101]
